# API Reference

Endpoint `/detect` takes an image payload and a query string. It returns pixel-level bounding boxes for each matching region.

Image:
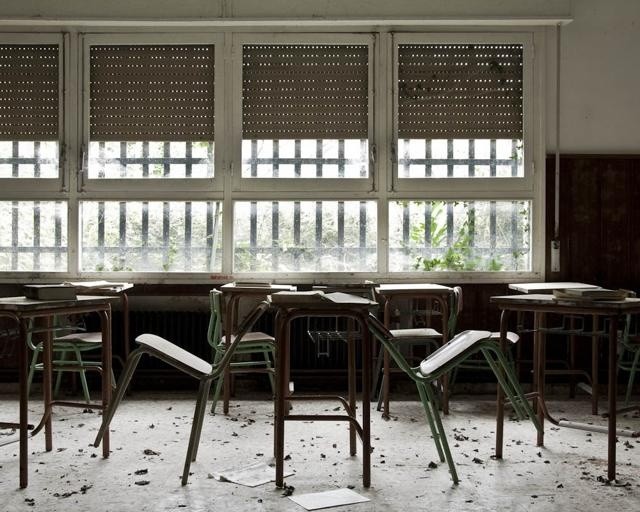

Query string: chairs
[92,296,272,490]
[202,279,296,419]
[605,292,639,412]
[360,308,546,489]
[441,279,526,419]
[373,281,470,425]
[22,305,122,419]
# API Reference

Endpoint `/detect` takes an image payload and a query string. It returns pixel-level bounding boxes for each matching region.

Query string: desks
[0,294,125,492]
[364,276,459,420]
[23,277,135,410]
[487,286,639,488]
[260,285,381,495]
[218,279,301,418]
[506,274,606,420]
